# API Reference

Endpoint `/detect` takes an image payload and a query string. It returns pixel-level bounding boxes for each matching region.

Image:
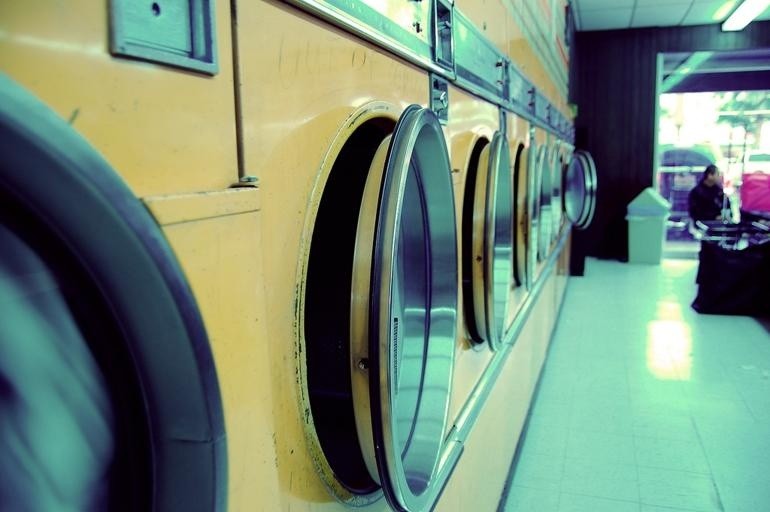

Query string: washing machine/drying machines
[1,0,598,512]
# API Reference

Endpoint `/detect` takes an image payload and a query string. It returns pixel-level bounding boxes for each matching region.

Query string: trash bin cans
[626,187,672,264]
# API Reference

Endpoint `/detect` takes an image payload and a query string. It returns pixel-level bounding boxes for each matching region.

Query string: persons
[685,165,734,227]
[739,169,770,219]
